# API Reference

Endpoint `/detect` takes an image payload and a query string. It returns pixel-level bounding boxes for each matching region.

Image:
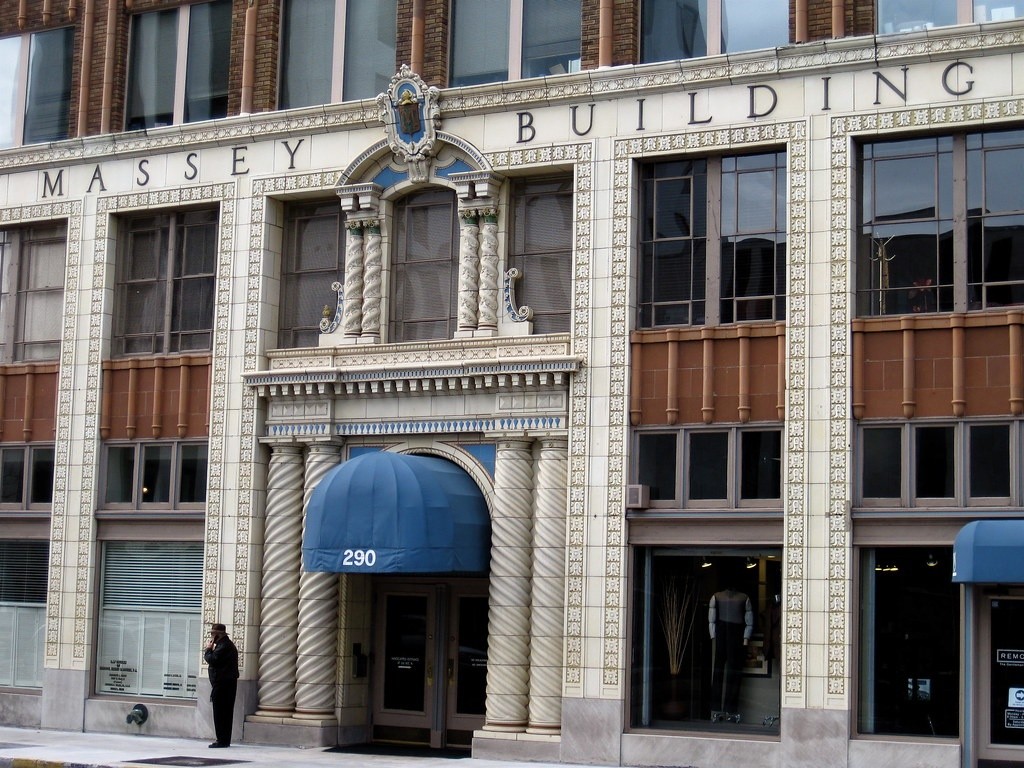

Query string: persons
[204,623,238,748]
[708,588,755,715]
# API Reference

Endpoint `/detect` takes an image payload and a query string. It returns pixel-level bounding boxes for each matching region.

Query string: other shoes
[208,740,230,748]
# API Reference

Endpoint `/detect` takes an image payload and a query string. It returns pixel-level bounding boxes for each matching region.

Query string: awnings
[299,452,490,574]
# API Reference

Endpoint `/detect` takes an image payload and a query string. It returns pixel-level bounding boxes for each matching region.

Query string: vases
[660,674,688,721]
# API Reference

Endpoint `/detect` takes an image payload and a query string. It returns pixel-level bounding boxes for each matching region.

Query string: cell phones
[213,635,219,643]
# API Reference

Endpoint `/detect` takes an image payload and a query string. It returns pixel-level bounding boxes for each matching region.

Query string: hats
[207,623,230,635]
